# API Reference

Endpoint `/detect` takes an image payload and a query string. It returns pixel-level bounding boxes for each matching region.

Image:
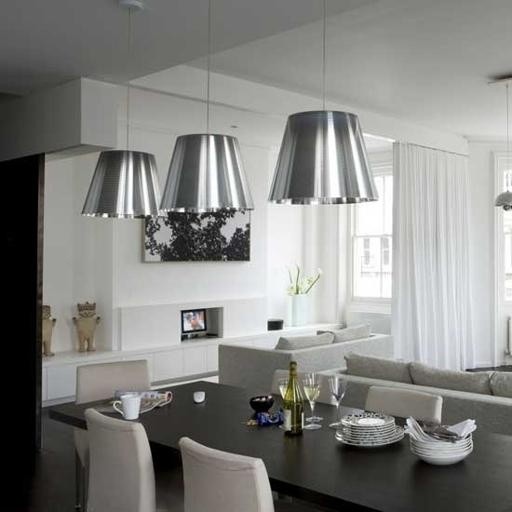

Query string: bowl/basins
[249,395,276,412]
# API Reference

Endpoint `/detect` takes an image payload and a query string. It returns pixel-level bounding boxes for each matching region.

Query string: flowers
[284,263,324,294]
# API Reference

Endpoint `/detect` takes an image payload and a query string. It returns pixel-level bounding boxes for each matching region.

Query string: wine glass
[302,371,323,430]
[326,374,349,428]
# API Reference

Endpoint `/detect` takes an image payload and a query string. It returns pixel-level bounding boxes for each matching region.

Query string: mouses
[193,392,205,403]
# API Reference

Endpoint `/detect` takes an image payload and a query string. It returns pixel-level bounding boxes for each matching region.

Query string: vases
[291,292,313,327]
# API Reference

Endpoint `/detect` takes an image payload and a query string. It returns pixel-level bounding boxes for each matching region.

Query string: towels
[404,416,478,443]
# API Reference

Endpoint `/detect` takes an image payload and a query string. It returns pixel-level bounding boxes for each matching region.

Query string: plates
[405,415,477,465]
[334,412,405,447]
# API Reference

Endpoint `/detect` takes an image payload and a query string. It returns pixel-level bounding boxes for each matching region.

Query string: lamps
[156,1,257,215]
[266,1,381,207]
[484,72,512,215]
[78,0,169,221]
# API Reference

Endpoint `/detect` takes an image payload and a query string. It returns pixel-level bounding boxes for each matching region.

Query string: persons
[184,312,194,330]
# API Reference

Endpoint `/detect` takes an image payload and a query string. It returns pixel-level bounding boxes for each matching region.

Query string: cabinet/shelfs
[39,349,114,406]
[126,325,342,387]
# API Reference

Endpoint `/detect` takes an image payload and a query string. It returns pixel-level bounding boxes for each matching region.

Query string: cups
[279,377,291,397]
[113,394,143,420]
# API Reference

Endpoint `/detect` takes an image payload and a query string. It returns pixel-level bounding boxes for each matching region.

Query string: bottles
[282,360,305,438]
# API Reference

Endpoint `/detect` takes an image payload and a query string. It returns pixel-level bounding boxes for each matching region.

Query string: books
[94,389,173,413]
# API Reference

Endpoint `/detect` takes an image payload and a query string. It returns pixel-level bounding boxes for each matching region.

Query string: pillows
[275,324,373,352]
[344,350,512,397]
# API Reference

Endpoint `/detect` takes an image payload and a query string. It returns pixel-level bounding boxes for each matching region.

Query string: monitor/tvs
[180,308,208,339]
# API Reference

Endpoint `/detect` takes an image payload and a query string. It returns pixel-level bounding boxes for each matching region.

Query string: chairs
[78,408,158,510]
[176,433,279,512]
[69,358,153,512]
[270,367,447,425]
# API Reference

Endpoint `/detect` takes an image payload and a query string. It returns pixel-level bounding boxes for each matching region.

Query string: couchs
[312,348,512,435]
[217,323,396,394]
